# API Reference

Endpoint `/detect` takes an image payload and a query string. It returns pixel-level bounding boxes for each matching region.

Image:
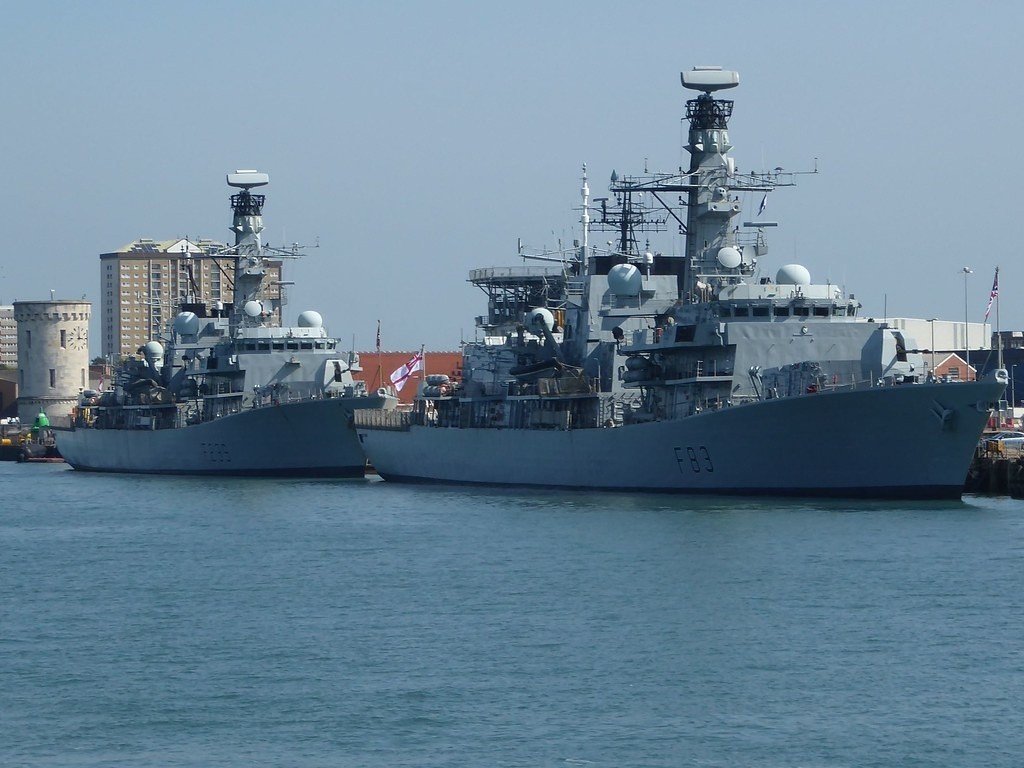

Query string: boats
[123,378,160,394]
[51,169,399,479]
[509,357,563,383]
[352,63,1010,502]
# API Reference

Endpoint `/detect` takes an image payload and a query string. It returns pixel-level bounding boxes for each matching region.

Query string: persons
[215,299,223,318]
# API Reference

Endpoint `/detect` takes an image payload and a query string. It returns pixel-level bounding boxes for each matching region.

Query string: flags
[376,323,380,350]
[984,269,998,321]
[389,346,425,392]
[98,373,104,391]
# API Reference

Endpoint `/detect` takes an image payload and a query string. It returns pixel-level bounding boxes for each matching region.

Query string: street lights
[964,267,974,382]
[926,318,938,376]
[1011,365,1017,418]
